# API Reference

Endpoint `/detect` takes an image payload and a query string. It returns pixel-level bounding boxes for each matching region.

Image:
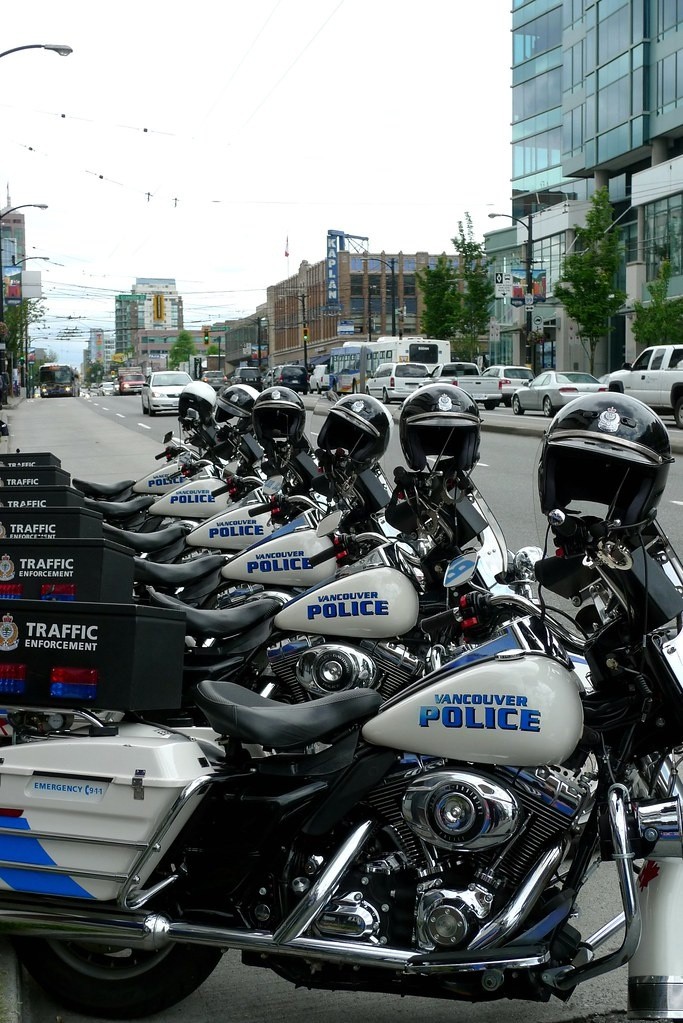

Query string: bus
[39,364,81,397]
[326,337,451,396]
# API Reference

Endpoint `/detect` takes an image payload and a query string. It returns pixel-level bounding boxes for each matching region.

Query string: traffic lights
[202,327,210,346]
[302,328,309,342]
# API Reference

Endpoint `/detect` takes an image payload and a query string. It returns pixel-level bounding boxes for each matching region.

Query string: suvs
[200,371,228,391]
[262,365,308,395]
[229,367,263,392]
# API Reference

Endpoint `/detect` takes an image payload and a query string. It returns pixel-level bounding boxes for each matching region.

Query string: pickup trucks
[418,361,502,410]
[603,344,683,429]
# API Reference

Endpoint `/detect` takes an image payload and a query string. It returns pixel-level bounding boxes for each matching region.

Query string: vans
[309,364,330,395]
[365,362,430,404]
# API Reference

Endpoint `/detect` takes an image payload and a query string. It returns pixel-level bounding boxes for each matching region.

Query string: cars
[141,371,194,417]
[479,365,534,407]
[511,371,608,416]
[80,375,146,396]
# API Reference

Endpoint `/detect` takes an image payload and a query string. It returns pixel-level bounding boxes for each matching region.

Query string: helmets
[178,381,216,419]
[251,386,306,441]
[215,384,258,430]
[398,382,480,471]
[317,394,394,464]
[539,392,669,526]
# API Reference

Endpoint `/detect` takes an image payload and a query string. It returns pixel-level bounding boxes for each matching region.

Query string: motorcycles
[0,392,683,1023]
[0,378,602,750]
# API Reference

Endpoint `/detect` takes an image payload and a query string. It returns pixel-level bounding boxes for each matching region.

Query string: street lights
[488,212,532,371]
[277,294,307,370]
[359,257,396,337]
